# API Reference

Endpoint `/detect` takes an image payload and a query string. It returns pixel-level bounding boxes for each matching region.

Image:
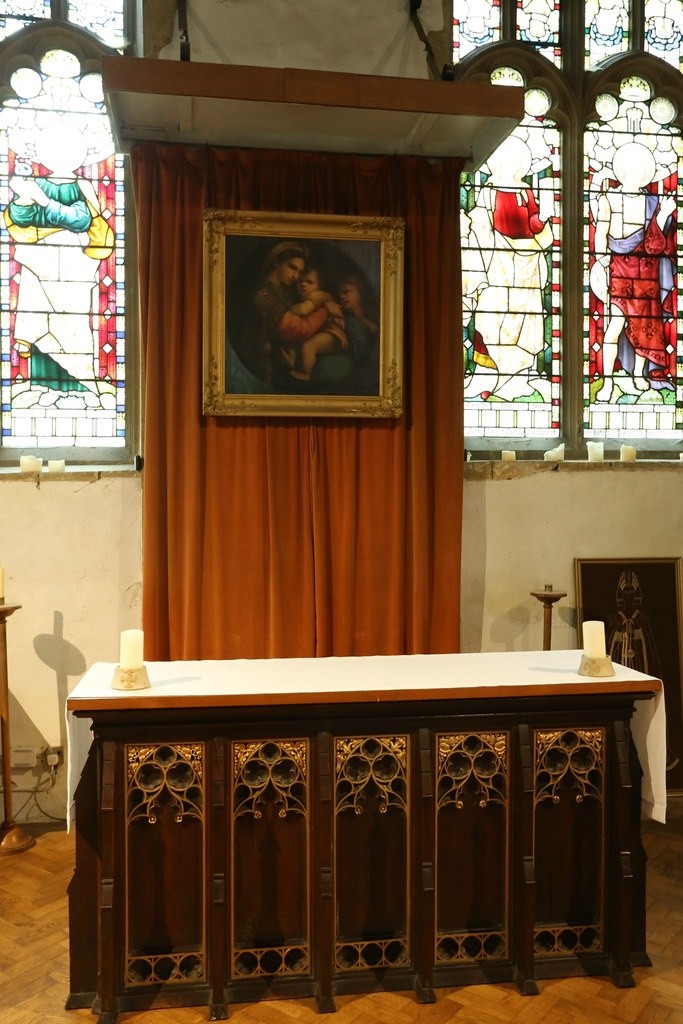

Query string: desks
[66,648,666,1023]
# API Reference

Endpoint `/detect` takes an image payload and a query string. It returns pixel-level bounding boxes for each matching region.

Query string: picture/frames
[201,207,403,419]
[573,558,683,796]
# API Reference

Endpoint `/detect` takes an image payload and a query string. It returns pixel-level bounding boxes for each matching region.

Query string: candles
[501,450,516,461]
[119,630,145,668]
[19,455,42,470]
[543,442,566,461]
[47,459,65,471]
[620,444,638,461]
[0,564,4,597]
[581,621,606,659]
[586,441,605,462]
